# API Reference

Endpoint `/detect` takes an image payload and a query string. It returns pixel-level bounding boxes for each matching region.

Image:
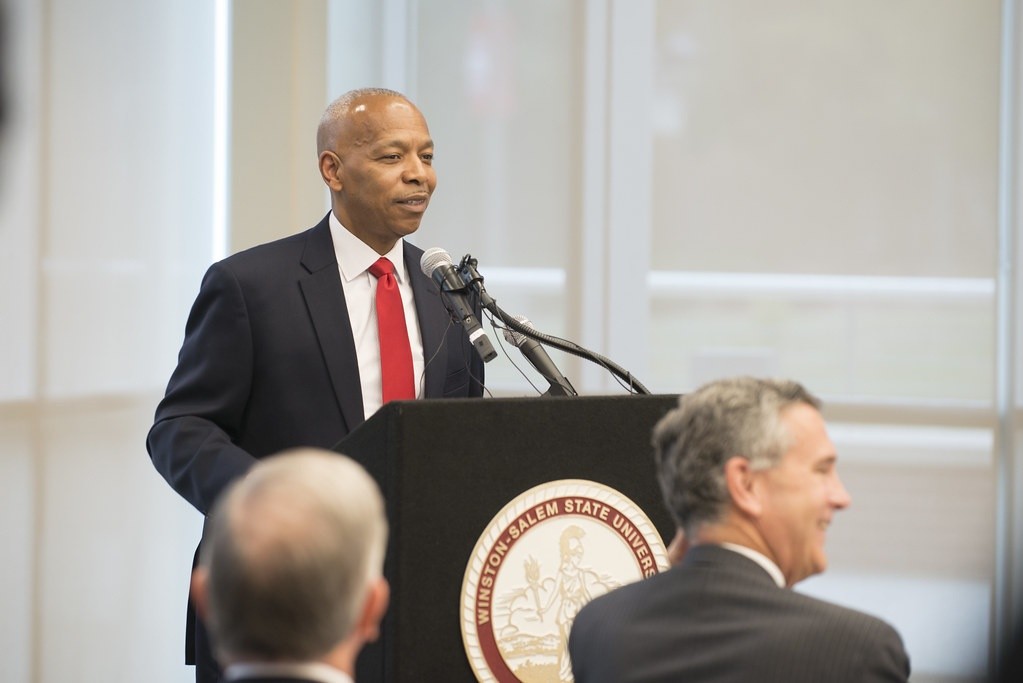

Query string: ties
[363,254,417,407]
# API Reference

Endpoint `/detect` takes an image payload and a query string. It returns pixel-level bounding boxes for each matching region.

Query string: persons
[145,87,486,665]
[568,378,910,683]
[191,447,389,683]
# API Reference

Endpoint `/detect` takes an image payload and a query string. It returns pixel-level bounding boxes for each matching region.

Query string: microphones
[503,314,577,399]
[420,248,498,363]
